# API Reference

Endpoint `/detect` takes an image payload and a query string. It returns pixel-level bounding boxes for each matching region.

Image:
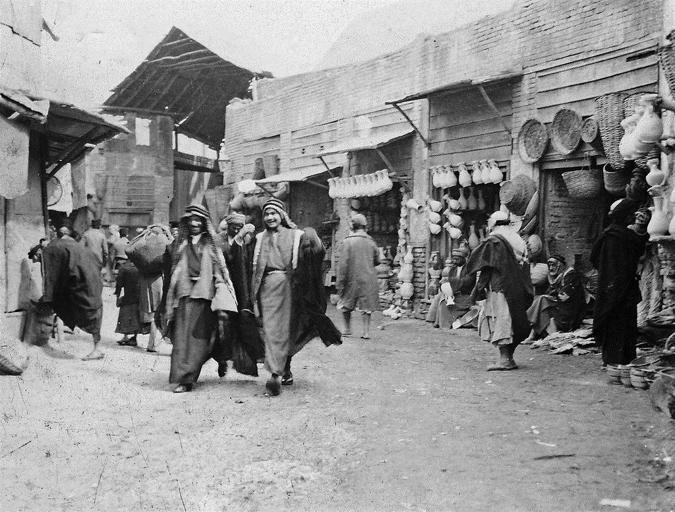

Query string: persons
[27,200,266,395]
[335,213,381,340]
[590,198,652,372]
[466,210,534,371]
[518,254,587,345]
[425,247,477,329]
[229,197,342,397]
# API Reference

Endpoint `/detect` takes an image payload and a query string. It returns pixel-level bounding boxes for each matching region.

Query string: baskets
[659,29,675,100]
[517,92,660,200]
[500,174,549,286]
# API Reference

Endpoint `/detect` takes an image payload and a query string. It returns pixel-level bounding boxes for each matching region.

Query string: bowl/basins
[608,353,664,390]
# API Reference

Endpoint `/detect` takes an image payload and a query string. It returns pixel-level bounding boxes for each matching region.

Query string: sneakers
[83,338,293,395]
